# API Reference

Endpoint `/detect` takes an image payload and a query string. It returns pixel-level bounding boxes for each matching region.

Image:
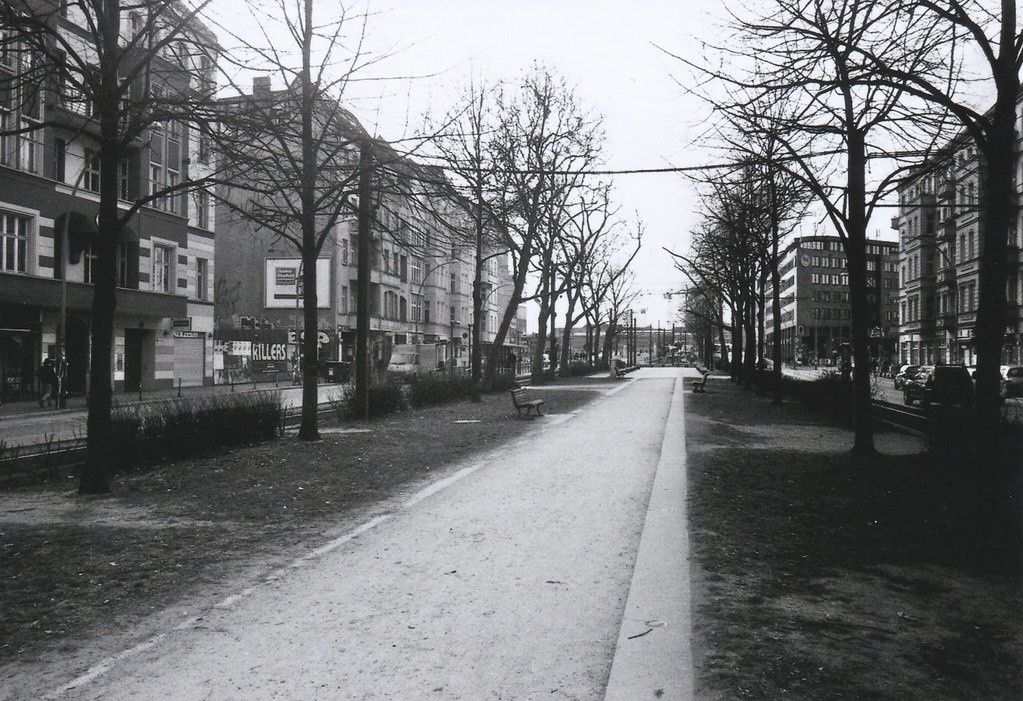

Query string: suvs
[903,365,974,409]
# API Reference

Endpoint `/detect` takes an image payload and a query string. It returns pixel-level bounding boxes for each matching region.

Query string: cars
[1000,364,1023,398]
[894,365,921,390]
[966,367,1006,406]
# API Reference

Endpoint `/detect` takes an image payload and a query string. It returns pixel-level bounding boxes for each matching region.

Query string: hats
[43,358,51,364]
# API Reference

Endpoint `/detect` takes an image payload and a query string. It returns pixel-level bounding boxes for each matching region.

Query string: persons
[39,358,57,408]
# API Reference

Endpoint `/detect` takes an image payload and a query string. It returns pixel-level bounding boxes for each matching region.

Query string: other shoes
[39,400,44,407]
[48,402,53,407]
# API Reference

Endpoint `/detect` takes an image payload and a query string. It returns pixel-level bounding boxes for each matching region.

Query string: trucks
[385,342,438,377]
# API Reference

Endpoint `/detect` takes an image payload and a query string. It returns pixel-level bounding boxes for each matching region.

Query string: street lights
[899,235,958,364]
[414,260,459,344]
[468,323,473,375]
[517,330,526,345]
[55,121,162,407]
[450,319,460,374]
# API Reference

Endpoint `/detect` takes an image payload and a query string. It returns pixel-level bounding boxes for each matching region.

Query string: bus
[479,340,529,374]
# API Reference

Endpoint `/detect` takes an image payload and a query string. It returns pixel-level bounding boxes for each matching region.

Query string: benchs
[692,372,708,393]
[507,387,547,421]
